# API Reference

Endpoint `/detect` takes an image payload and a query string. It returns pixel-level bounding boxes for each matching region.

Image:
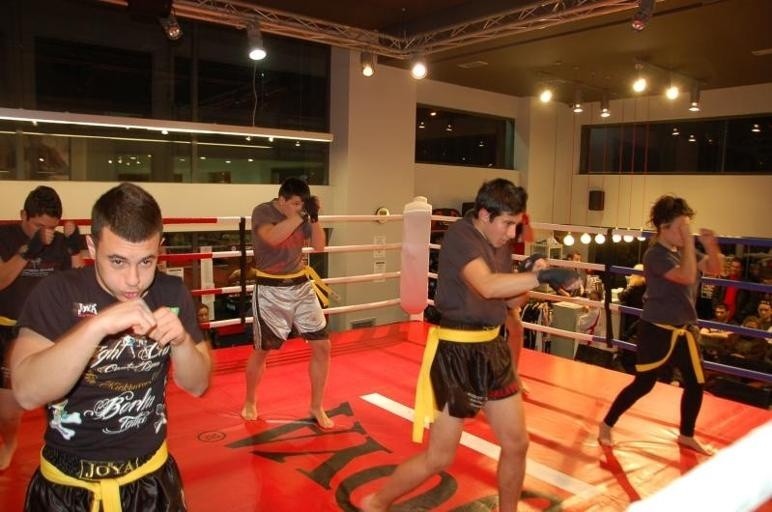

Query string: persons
[10,183,212,512]
[196,303,219,349]
[693,258,772,391]
[361,178,582,512]
[0,186,85,470]
[598,192,722,456]
[493,187,648,394]
[241,179,335,430]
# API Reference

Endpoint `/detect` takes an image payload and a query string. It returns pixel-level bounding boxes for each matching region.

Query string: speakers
[589,191,604,210]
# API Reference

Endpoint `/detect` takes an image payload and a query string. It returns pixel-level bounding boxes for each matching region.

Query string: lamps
[407,54,430,82]
[631,1,654,32]
[156,10,376,79]
[569,88,610,118]
[688,86,701,113]
